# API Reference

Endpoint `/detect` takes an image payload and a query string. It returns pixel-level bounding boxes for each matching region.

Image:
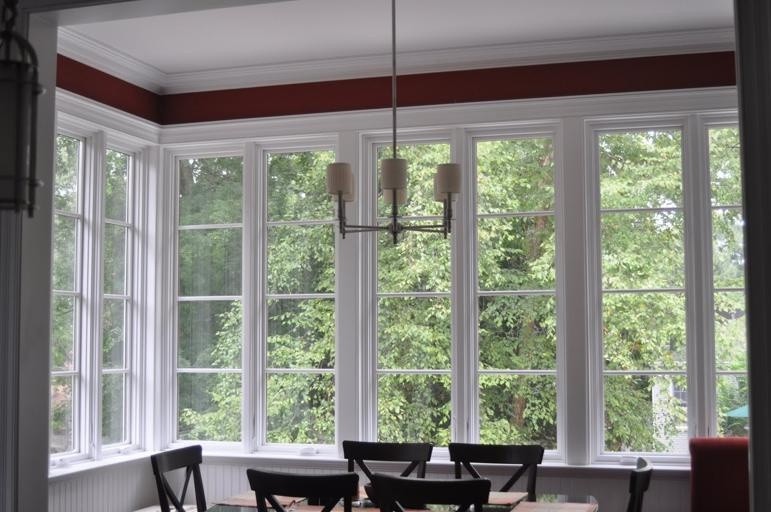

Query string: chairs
[243,466,362,512]
[340,440,435,496]
[151,442,209,511]
[367,471,493,511]
[446,441,546,501]
[625,455,657,511]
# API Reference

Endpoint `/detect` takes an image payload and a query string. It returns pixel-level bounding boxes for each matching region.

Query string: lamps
[324,2,463,245]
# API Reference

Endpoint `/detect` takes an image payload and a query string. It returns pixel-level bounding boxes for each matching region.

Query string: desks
[213,484,600,510]
